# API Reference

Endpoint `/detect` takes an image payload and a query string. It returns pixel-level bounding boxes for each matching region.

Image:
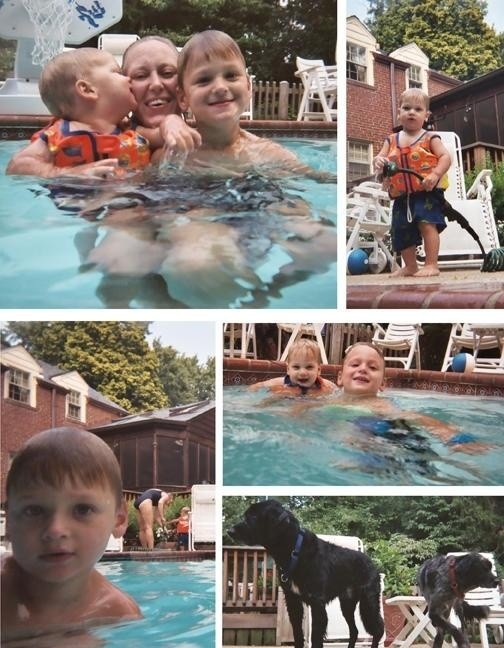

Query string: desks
[386,594,450,647]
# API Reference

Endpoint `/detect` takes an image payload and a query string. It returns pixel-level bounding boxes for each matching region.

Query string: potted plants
[369,528,445,647]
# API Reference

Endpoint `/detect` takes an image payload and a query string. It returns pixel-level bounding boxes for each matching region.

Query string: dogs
[226,497,388,647]
[415,548,502,648]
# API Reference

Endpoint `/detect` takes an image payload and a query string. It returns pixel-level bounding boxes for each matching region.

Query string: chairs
[346,176,400,271]
[274,323,330,364]
[292,55,338,123]
[370,322,425,369]
[309,531,387,646]
[438,323,504,373]
[408,129,500,270]
[96,32,139,65]
[184,483,215,551]
[240,67,256,122]
[447,550,504,647]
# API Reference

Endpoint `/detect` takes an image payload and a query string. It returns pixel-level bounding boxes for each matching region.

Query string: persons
[8,28,337,304]
[168,507,189,550]
[374,89,451,278]
[242,328,338,413]
[0,427,143,648]
[290,342,495,457]
[134,490,175,549]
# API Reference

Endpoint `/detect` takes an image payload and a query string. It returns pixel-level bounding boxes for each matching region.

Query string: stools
[467,323,504,375]
[223,322,257,359]
